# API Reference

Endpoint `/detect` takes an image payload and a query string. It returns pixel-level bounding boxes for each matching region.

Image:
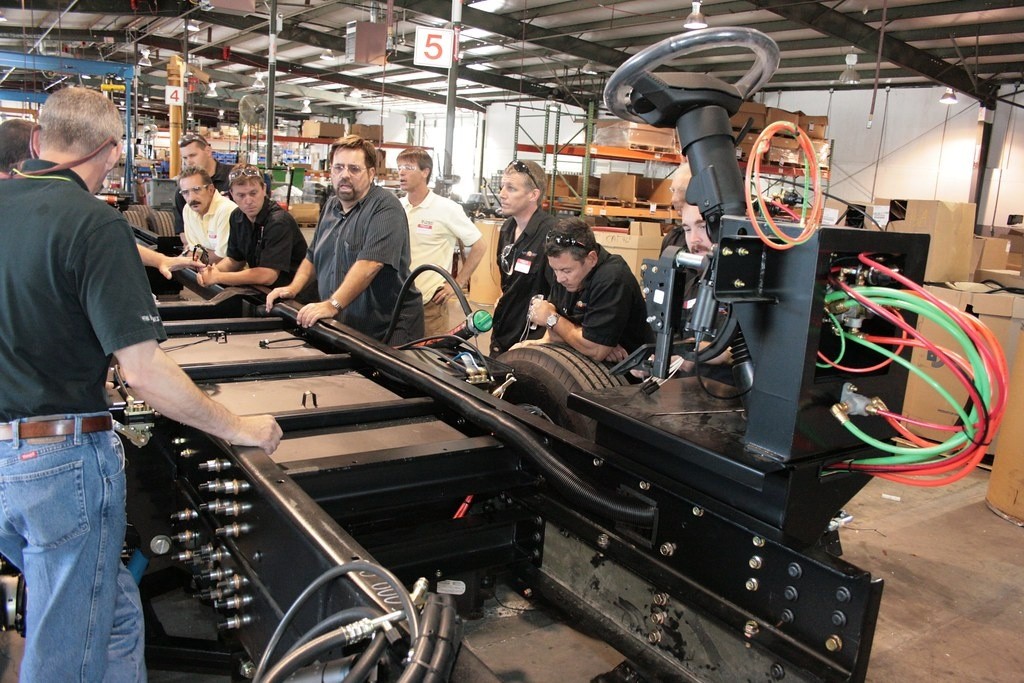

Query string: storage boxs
[293,99,1024,470]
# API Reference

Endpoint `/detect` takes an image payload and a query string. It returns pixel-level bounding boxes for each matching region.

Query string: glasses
[397,164,423,173]
[177,134,207,146]
[508,159,540,189]
[329,163,369,177]
[229,165,262,186]
[545,229,591,252]
[501,243,517,276]
[179,183,210,197]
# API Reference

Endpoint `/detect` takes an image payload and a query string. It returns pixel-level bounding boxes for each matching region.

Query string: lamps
[78,12,361,122]
[684,0,708,29]
[582,59,598,75]
[940,87,958,104]
[839,44,862,83]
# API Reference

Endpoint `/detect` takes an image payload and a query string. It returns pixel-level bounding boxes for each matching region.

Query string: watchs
[546,313,560,328]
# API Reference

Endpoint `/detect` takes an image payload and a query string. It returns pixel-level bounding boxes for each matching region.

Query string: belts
[0,416,113,441]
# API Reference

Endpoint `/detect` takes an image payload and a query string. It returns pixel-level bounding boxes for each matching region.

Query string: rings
[442,294,445,297]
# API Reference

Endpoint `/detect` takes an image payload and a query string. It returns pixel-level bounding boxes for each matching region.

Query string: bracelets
[329,298,342,310]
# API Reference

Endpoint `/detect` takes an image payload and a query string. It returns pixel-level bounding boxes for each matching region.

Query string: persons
[198,164,318,307]
[530,216,657,385]
[175,135,235,217]
[0,119,205,280]
[659,163,731,375]
[0,86,283,682]
[266,136,425,347]
[396,148,487,338]
[178,166,238,265]
[488,159,557,358]
